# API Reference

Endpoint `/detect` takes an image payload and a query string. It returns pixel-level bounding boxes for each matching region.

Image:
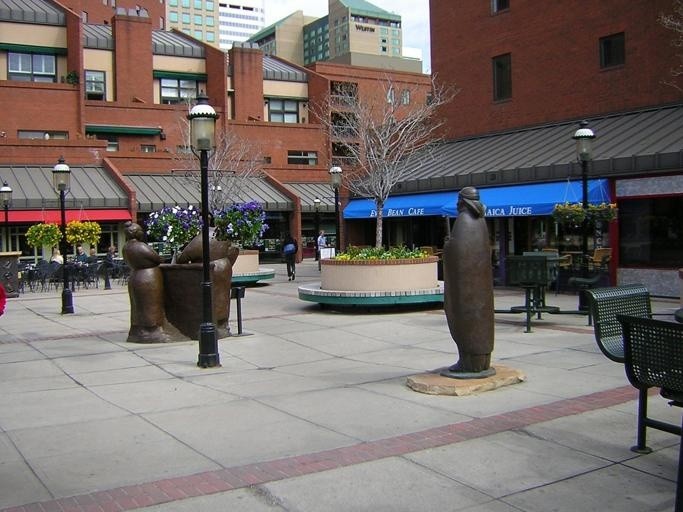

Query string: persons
[317,230,327,252]
[282,233,298,281]
[441,187,494,373]
[50,246,115,264]
[122,221,165,328]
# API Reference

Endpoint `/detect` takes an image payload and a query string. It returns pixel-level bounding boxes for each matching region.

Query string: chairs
[615,313,683,512]
[567,254,610,326]
[584,282,676,454]
[523,251,559,319]
[505,255,548,333]
[589,247,611,269]
[18,259,130,294]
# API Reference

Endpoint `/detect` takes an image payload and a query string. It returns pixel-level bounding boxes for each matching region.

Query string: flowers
[587,202,619,227]
[143,203,203,249]
[212,200,271,248]
[552,200,583,230]
[331,242,430,261]
[67,222,100,247]
[27,224,61,249]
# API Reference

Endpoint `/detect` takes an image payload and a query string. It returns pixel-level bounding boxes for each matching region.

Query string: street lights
[211,184,220,193]
[51,155,74,315]
[313,196,320,260]
[327,167,342,255]
[570,120,596,312]
[0,180,12,251]
[186,90,220,371]
